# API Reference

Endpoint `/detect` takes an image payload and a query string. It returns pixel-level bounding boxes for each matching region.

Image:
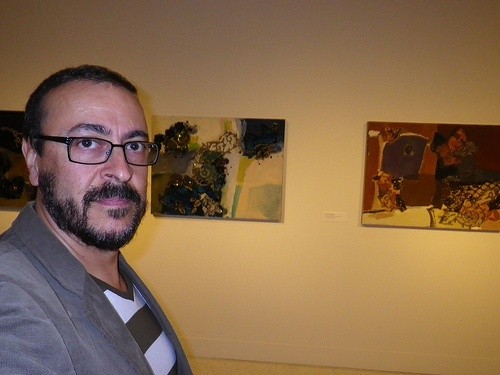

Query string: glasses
[31,134,159,166]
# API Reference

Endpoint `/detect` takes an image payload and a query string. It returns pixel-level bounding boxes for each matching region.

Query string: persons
[0,65,194,375]
